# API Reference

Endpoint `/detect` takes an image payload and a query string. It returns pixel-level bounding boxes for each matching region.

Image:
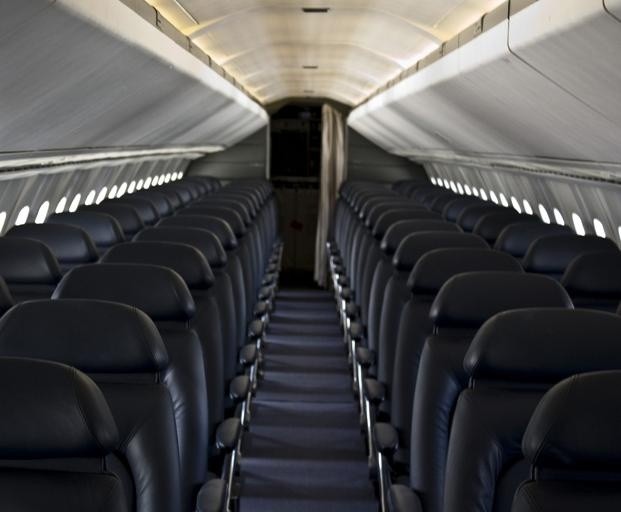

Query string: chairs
[1,174,287,512]
[325,179,621,511]
